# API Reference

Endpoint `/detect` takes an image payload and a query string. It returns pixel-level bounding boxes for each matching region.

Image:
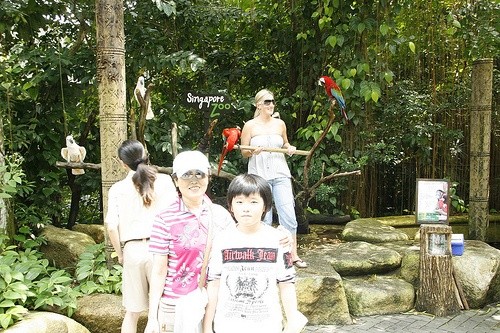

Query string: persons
[146,148,293,333]
[109,137,180,333]
[204,173,302,333]
[239,89,307,268]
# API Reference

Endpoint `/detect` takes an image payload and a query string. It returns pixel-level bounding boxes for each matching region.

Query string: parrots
[60,135,87,175]
[134,76,155,120]
[217,125,242,176]
[318,75,349,121]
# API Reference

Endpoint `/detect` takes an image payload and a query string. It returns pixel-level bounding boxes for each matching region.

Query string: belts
[123,237,152,246]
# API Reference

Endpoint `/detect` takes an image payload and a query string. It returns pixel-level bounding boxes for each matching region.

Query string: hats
[173,150,211,178]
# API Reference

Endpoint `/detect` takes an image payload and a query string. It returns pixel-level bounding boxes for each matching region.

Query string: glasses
[179,170,205,179]
[257,99,275,105]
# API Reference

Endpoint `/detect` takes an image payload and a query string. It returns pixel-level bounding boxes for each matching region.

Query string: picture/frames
[416,178,450,224]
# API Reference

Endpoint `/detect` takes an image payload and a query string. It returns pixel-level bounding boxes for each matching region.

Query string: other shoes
[293,259,307,268]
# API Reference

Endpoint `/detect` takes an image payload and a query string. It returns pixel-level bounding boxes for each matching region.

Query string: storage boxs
[451,233,464,255]
